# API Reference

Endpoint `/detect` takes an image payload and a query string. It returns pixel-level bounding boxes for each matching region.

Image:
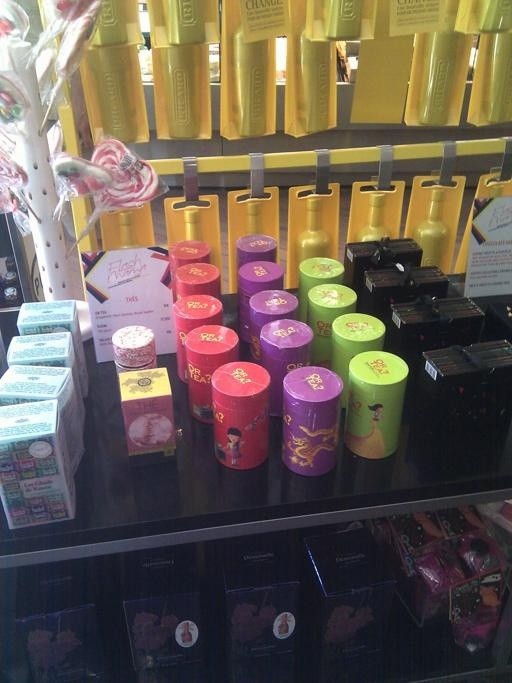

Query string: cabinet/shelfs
[0,273,512,682]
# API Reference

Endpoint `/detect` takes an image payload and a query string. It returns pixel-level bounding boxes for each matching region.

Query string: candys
[0,0,105,223]
[69,139,170,259]
[50,154,113,221]
